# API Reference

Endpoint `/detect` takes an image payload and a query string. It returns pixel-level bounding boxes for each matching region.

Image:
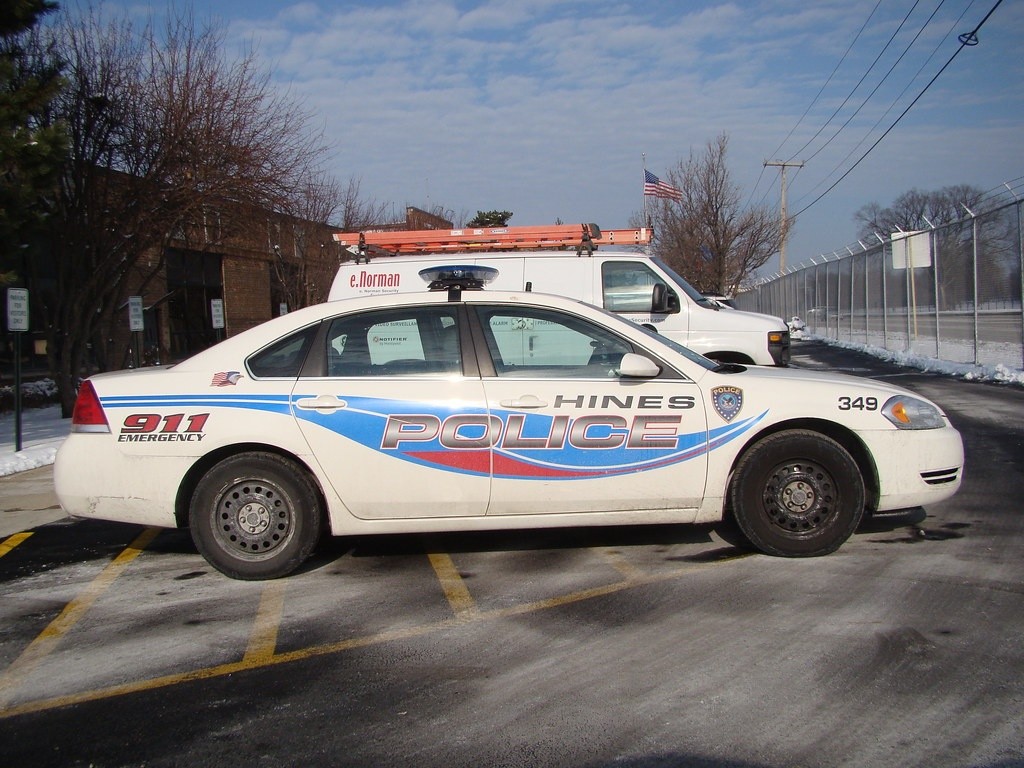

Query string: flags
[644,170,682,203]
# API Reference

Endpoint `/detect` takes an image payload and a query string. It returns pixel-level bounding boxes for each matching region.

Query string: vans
[324,224,790,371]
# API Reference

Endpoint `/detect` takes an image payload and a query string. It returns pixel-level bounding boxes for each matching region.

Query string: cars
[808,308,843,320]
[51,270,966,582]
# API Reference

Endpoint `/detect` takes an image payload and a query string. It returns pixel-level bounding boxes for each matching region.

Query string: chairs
[439,326,461,371]
